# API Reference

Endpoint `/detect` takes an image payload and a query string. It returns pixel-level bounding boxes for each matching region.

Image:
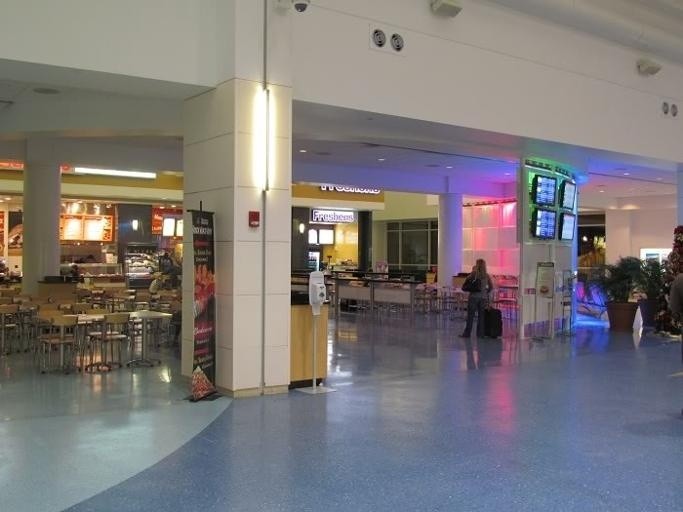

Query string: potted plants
[584,252,673,333]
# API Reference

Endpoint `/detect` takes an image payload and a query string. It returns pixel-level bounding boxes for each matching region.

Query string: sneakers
[458,334,471,338]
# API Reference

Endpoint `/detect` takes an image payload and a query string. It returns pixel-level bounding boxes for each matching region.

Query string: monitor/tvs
[531,174,557,206]
[559,179,577,211]
[531,207,557,240]
[559,212,575,240]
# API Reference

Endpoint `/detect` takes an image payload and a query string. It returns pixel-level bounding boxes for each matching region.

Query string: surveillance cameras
[291,0,310,13]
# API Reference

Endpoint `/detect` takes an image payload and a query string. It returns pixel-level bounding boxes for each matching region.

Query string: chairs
[1,283,183,379]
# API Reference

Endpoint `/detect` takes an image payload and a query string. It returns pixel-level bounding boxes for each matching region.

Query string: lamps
[431,1,465,17]
[637,57,664,77]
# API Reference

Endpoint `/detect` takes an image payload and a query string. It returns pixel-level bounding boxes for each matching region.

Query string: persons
[457,257,491,337]
[667,272,682,364]
[462,335,491,415]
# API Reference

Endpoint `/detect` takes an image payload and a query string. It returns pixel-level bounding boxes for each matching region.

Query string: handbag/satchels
[461,269,481,293]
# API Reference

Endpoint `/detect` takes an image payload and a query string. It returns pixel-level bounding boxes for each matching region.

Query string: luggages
[484,306,503,339]
